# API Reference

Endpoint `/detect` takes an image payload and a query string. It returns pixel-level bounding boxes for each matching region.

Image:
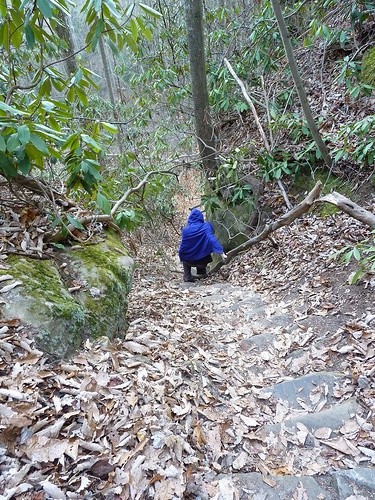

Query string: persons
[178,208,228,282]
